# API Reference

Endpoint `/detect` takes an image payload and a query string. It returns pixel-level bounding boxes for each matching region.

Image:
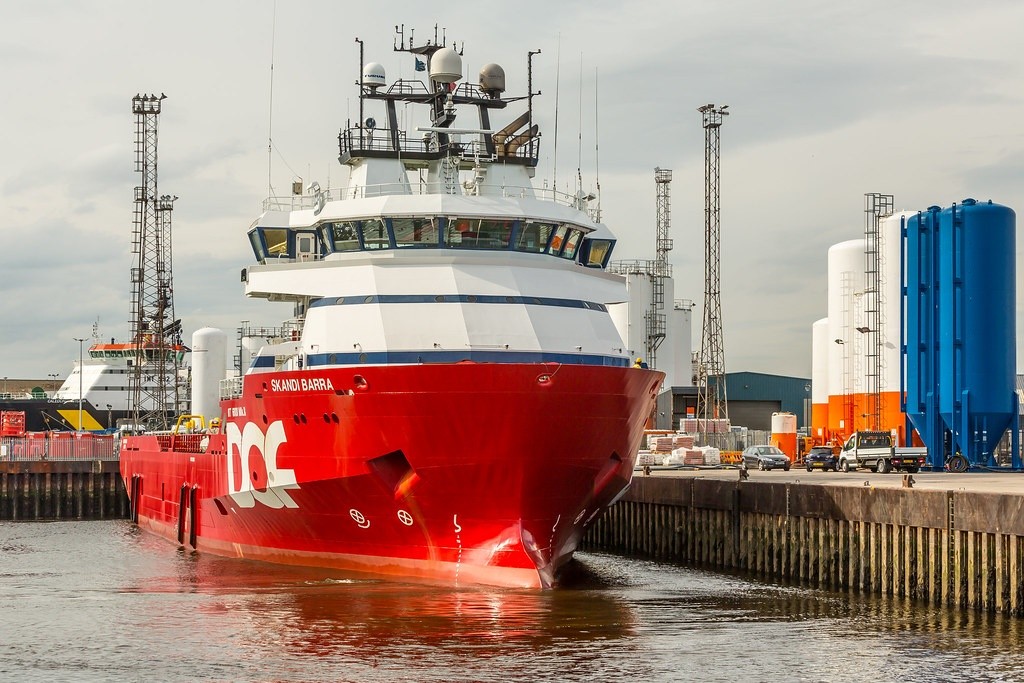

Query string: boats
[120,19,666,591]
[1,320,195,435]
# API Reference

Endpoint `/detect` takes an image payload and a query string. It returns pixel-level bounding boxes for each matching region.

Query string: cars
[742,446,790,471]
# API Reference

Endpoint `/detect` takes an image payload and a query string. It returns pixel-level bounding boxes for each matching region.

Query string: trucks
[838,430,927,472]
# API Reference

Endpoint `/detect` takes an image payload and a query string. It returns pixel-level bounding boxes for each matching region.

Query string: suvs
[805,446,844,472]
